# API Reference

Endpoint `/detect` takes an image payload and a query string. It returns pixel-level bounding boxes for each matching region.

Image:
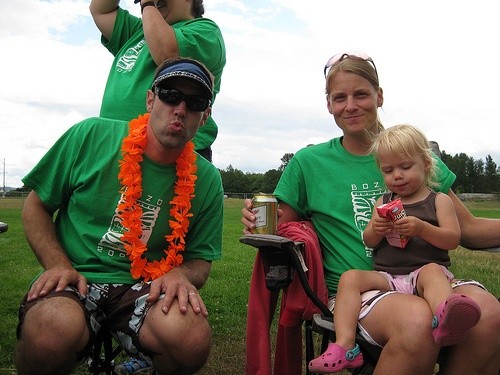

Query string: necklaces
[122,114,196,283]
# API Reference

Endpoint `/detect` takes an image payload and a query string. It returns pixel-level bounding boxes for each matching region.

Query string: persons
[15,58,223,375]
[309,126,482,374]
[89,0,225,162]
[241,54,500,375]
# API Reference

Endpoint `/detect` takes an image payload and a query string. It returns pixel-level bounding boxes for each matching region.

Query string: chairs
[240,142,439,375]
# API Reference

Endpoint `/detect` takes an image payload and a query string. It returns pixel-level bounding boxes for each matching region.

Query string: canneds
[249,192,278,235]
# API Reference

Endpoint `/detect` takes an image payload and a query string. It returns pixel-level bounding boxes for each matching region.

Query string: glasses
[324,51,378,80]
[153,84,212,112]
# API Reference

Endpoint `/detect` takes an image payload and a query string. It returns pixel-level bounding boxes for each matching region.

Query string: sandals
[113,354,153,375]
[432,295,481,346]
[308,342,363,372]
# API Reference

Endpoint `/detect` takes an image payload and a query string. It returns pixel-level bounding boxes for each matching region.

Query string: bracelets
[141,1,157,14]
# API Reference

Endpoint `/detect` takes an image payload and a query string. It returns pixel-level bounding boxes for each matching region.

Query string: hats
[152,63,213,101]
